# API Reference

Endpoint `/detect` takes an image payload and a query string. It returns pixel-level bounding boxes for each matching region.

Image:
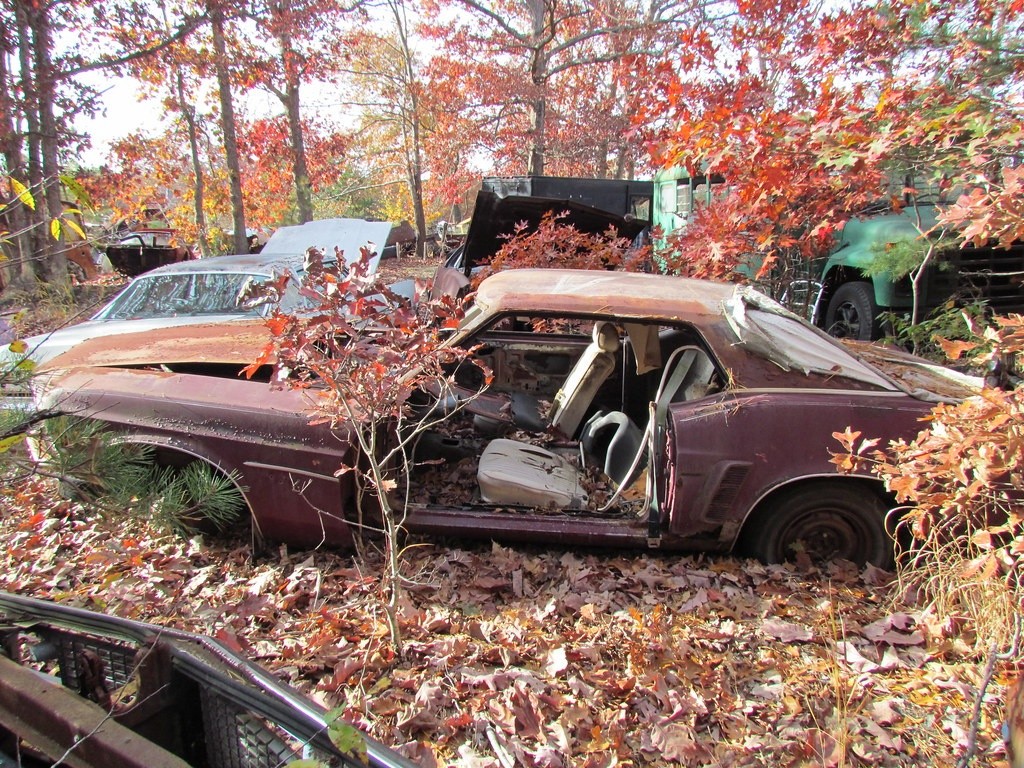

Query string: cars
[0,218,416,410]
[430,175,655,311]
[27,268,1024,577]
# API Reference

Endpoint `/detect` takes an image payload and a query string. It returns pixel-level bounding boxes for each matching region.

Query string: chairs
[469,345,716,508]
[473,321,620,438]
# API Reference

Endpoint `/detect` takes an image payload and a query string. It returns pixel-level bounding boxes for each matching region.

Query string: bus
[653,151,1024,342]
